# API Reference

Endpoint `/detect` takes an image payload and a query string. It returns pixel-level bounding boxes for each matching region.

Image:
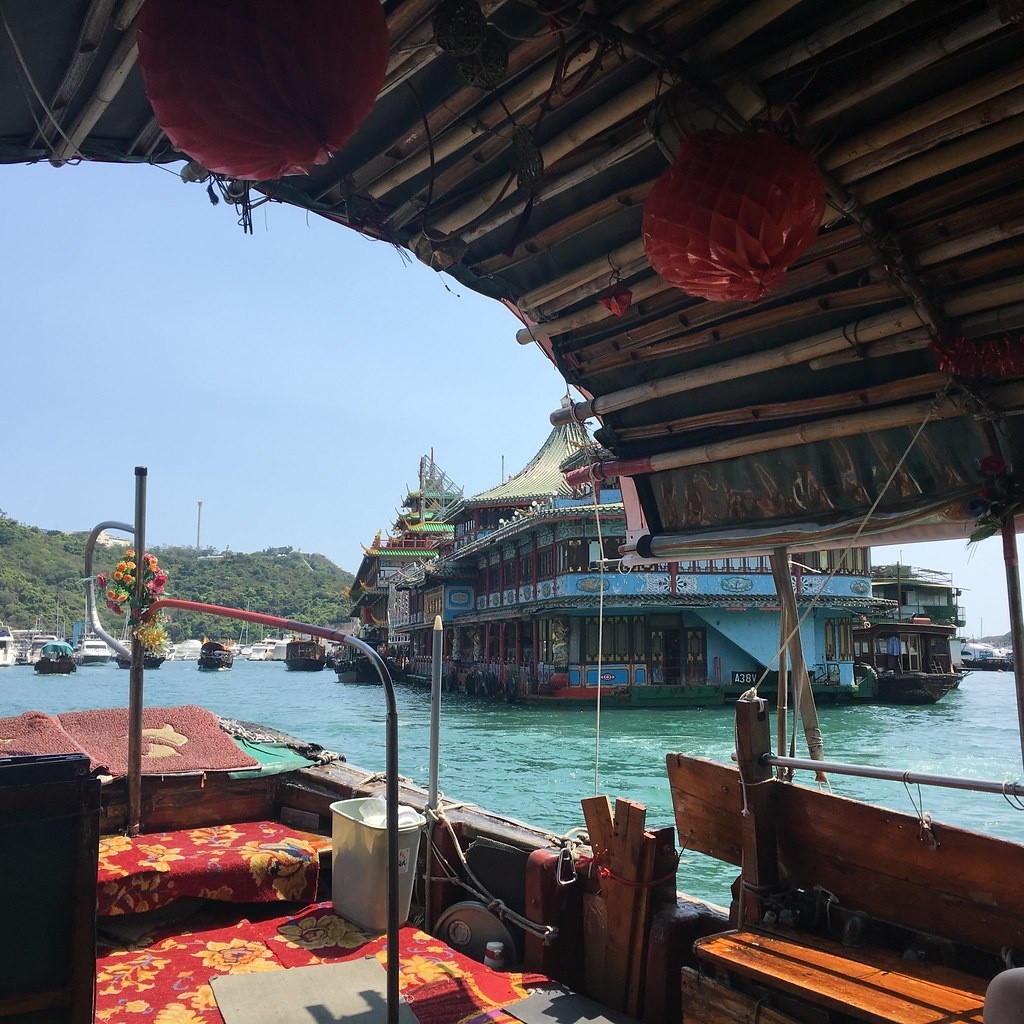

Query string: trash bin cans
[329,795,427,933]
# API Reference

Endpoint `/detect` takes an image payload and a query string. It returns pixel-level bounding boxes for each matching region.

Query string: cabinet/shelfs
[0,777,103,1024]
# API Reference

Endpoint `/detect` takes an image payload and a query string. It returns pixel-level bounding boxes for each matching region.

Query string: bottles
[484,942,504,970]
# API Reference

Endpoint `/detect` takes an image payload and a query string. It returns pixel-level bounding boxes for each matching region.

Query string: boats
[197,636,236,672]
[336,622,405,684]
[34,641,77,676]
[116,645,166,669]
[284,632,327,671]
[0,622,18,667]
[247,644,275,661]
[851,560,974,704]
[77,641,112,665]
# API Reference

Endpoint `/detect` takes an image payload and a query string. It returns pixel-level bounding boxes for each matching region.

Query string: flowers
[98,549,175,657]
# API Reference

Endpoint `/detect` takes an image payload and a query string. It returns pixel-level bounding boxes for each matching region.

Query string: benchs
[680,921,987,1024]
[96,771,340,915]
